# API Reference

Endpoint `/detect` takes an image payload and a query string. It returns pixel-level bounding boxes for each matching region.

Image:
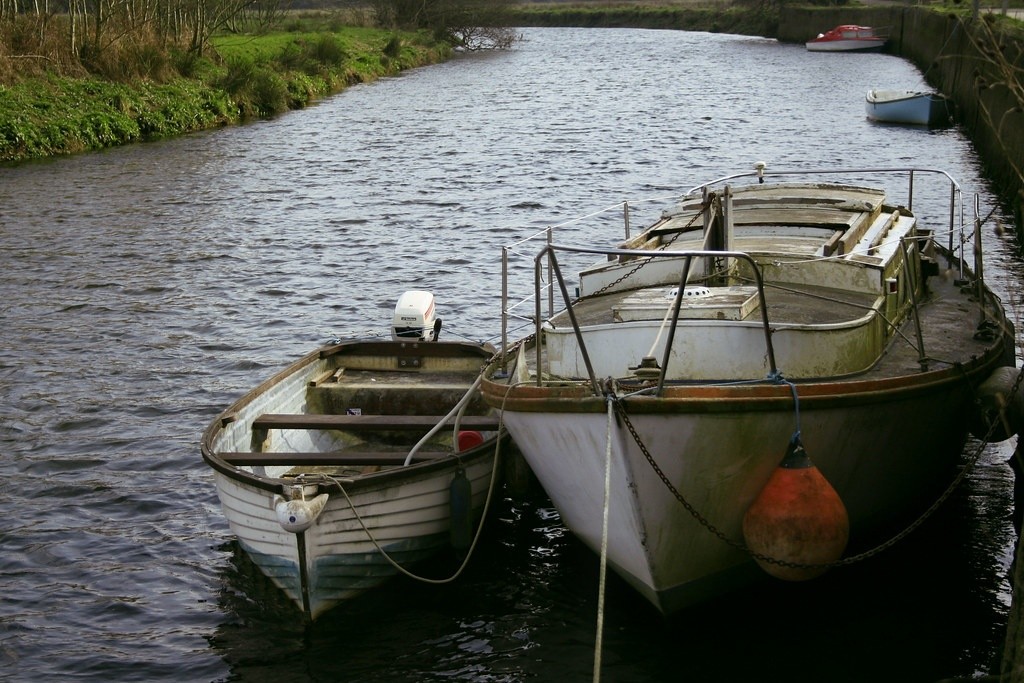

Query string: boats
[479,162,1015,618]
[200,287,517,622]
[805,24,887,51]
[865,87,955,126]
